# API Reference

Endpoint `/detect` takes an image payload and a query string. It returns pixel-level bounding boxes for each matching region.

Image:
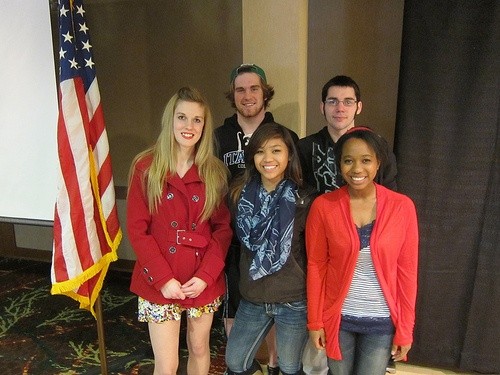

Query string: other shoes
[384,356,396,372]
[267,363,280,375]
[226,357,264,375]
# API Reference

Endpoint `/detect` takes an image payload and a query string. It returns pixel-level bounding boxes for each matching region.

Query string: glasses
[323,98,358,106]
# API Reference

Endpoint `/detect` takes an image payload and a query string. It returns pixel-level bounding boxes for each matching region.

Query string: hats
[230,63,266,82]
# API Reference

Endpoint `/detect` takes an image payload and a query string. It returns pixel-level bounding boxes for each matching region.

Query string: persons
[301,76,399,372]
[213,63,306,375]
[126,86,233,375]
[225,124,313,375]
[304,126,420,375]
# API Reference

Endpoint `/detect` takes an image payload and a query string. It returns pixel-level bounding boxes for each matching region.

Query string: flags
[50,0,122,321]
